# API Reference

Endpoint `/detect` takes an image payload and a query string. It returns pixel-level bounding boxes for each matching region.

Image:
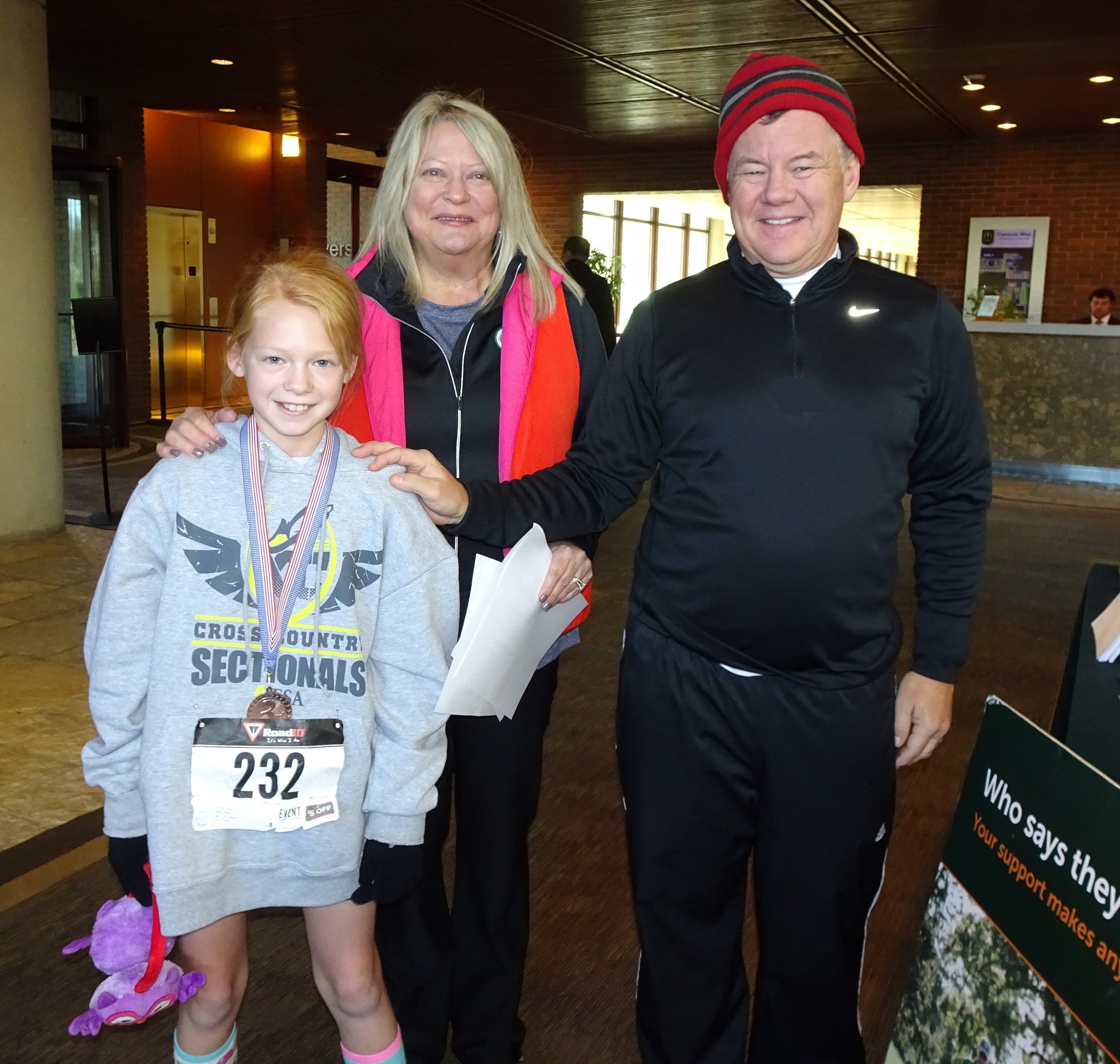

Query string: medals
[246,690,294,720]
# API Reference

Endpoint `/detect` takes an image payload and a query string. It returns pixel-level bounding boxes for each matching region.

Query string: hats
[714,52,866,206]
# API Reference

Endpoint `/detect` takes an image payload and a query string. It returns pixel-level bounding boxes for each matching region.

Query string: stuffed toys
[61,863,207,1037]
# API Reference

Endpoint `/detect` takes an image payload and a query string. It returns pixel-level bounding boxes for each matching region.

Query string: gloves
[350,839,425,907]
[107,832,157,911]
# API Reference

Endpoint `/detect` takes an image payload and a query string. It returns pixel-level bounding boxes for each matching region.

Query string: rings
[572,577,586,590]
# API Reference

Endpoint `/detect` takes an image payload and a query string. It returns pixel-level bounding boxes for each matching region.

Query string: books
[1090,594,1120,662]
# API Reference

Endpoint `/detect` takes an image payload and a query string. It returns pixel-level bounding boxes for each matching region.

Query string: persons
[1071,287,1120,325]
[153,97,615,1064]
[346,55,990,1064]
[78,250,460,1064]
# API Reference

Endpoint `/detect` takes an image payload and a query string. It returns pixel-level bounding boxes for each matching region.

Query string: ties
[1097,319,1102,325]
